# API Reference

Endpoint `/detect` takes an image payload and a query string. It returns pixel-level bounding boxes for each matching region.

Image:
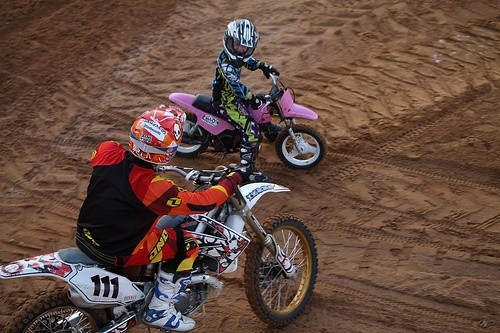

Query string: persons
[210,17,279,184]
[74,104,242,332]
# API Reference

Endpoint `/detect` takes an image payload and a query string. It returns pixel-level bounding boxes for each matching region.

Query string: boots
[260,121,284,143]
[142,262,197,333]
[239,141,271,183]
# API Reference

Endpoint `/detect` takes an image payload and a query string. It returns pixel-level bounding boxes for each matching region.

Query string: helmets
[128,105,187,165]
[222,18,259,68]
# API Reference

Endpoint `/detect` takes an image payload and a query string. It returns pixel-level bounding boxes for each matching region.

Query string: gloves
[248,93,265,110]
[259,62,280,79]
[230,163,253,184]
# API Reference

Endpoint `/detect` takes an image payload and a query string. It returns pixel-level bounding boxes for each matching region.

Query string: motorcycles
[1,146,320,333]
[168,72,328,170]
